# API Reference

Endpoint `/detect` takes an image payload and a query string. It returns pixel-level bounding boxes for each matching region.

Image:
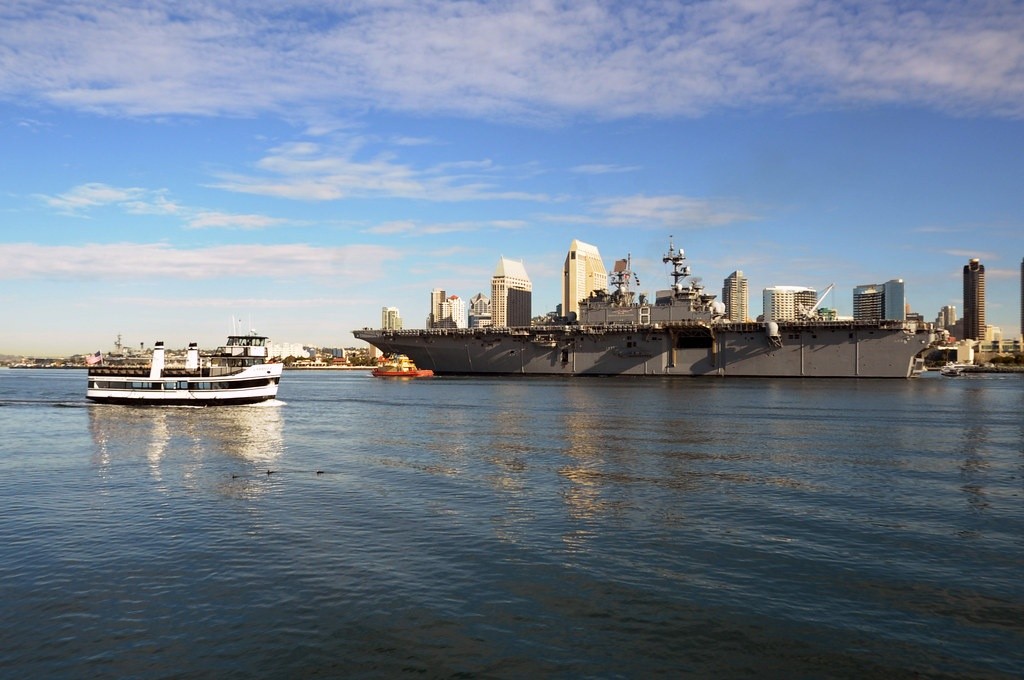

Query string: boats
[941,365,965,376]
[86,314,284,406]
[372,354,435,379]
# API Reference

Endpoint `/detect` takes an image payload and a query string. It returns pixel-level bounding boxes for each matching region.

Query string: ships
[352,235,949,379]
